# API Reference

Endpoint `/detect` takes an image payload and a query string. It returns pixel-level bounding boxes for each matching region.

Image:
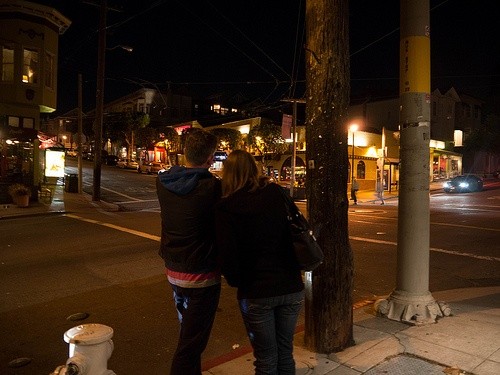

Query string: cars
[66,148,173,175]
[442,175,484,194]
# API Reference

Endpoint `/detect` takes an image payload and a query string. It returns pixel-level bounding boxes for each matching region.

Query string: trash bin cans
[280,182,305,198]
[64,173,78,193]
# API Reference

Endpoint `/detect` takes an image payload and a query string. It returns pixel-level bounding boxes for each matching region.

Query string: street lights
[91,45,132,200]
[350,123,358,199]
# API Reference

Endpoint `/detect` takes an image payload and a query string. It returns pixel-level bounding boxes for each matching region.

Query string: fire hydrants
[59,323,118,375]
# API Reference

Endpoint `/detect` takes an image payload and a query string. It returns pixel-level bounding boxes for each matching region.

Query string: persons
[350,176,358,204]
[376,179,385,205]
[156,131,228,375]
[219,150,306,375]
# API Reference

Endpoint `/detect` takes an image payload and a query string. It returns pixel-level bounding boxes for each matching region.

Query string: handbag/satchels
[276,184,325,271]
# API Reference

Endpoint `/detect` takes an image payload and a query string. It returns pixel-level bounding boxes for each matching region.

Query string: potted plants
[9,184,30,207]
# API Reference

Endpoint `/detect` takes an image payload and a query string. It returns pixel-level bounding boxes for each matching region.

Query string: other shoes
[381,203,384,205]
